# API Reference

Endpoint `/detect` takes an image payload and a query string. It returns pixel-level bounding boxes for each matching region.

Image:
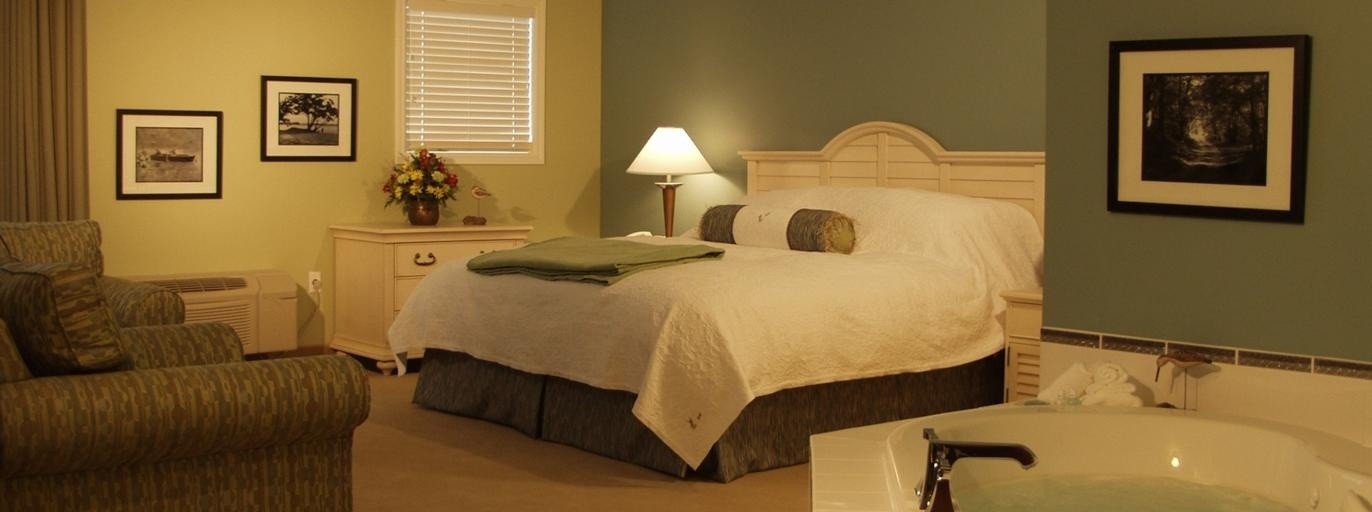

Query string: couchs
[1,219,371,512]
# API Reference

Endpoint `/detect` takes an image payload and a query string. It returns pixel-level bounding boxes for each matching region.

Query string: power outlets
[308,271,324,295]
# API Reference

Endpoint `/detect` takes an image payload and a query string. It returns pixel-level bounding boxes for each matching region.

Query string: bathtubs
[879,409,1371,512]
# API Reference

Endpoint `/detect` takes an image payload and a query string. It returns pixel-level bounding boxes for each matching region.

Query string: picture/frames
[260,75,359,161]
[114,109,224,201]
[1106,33,1314,225]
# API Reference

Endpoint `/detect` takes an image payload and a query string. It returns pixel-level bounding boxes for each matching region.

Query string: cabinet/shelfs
[329,219,533,376]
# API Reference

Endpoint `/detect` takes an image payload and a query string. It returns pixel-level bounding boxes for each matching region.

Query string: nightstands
[999,287,1043,406]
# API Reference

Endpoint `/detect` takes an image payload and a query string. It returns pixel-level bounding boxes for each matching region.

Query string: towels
[1080,382,1136,406]
[1086,364,1126,396]
[1037,361,1093,405]
[1106,395,1144,408]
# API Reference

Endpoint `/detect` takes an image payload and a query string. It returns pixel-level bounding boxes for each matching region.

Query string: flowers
[382,148,462,209]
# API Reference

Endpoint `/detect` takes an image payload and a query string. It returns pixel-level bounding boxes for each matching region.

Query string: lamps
[625,127,714,238]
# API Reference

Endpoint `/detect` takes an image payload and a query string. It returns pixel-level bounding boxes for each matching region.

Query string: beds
[388,121,1045,482]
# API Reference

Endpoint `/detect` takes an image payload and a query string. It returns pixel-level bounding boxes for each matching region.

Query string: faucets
[917,427,1036,512]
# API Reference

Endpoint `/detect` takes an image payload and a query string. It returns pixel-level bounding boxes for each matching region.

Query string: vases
[407,193,439,226]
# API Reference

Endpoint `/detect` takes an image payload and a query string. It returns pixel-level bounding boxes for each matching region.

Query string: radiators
[121,274,300,356]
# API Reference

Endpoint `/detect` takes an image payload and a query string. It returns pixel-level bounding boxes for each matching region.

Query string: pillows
[701,205,857,253]
[99,276,185,329]
[118,322,245,370]
[1,254,130,375]
